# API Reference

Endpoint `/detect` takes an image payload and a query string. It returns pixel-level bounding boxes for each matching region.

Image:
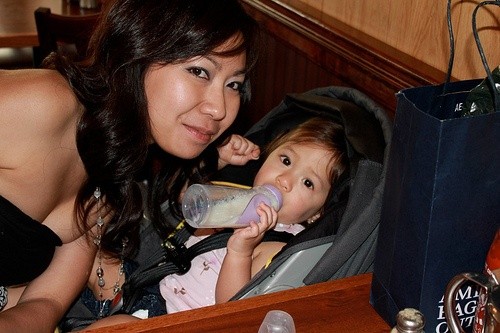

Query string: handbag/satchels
[369,1,498,331]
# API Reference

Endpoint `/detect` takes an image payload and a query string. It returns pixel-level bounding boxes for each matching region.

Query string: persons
[77,115,357,332]
[0,1,261,332]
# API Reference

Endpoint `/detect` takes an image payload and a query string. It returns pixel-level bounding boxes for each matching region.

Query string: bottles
[182,178,282,231]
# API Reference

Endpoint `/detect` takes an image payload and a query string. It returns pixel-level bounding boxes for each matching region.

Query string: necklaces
[94,180,128,322]
